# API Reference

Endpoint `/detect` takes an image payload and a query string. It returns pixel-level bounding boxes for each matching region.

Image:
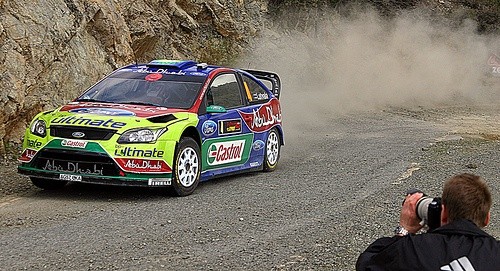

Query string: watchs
[393,225,410,236]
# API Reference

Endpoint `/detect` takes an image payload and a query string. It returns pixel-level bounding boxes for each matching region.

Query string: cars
[16,58,283,197]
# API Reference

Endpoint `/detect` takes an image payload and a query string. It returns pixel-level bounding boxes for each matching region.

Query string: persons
[356,171,500,271]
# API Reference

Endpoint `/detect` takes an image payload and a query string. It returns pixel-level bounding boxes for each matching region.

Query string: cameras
[401,188,443,228]
